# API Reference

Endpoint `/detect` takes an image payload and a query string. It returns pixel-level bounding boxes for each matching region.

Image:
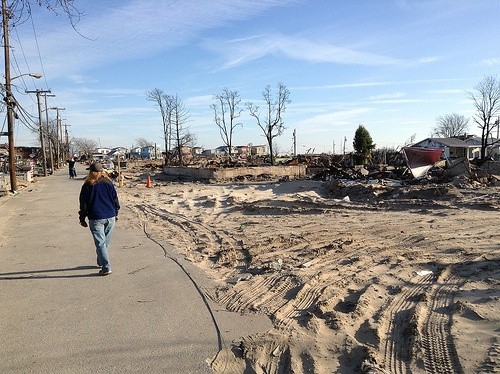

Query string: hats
[85,163,103,172]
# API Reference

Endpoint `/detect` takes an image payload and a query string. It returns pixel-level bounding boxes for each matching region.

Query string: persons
[66,158,77,179]
[79,163,120,275]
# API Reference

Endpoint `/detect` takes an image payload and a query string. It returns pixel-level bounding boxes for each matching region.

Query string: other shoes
[99,269,112,274]
[97,262,102,266]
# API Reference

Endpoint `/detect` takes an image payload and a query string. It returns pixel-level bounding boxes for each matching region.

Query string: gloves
[80,220,88,227]
